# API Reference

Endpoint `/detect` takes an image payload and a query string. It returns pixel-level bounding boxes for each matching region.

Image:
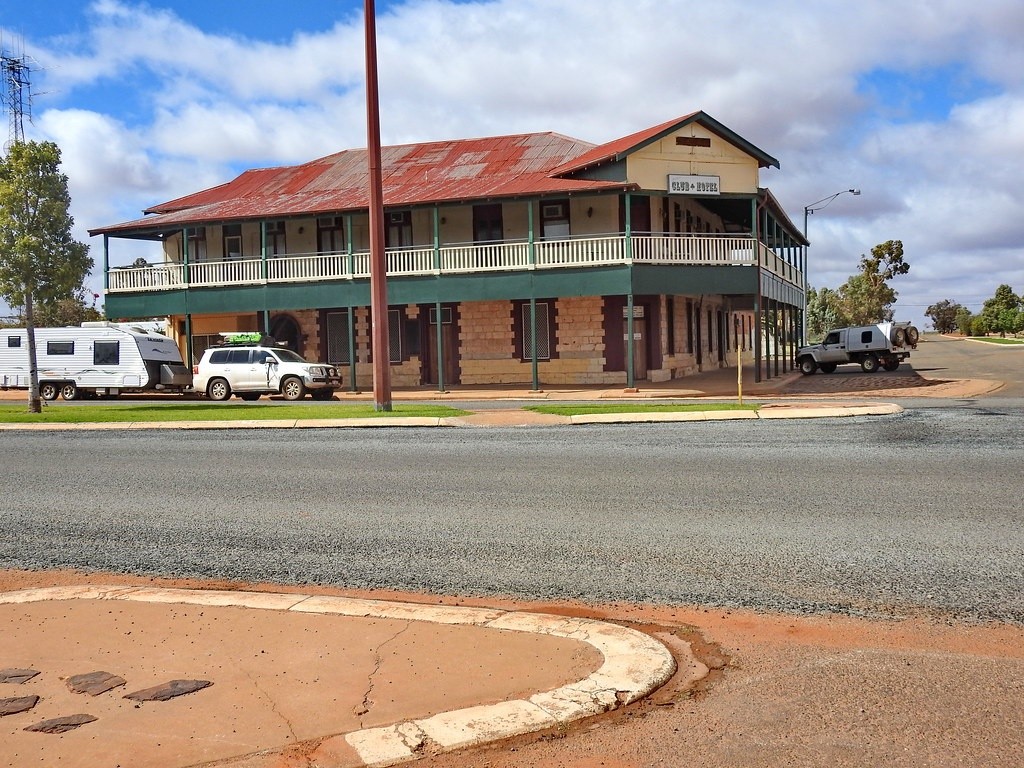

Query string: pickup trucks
[795,322,919,374]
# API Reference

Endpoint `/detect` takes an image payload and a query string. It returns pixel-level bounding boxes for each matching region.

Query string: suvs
[193,342,343,401]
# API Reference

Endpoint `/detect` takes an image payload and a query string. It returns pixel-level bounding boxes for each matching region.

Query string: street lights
[803,189,861,345]
